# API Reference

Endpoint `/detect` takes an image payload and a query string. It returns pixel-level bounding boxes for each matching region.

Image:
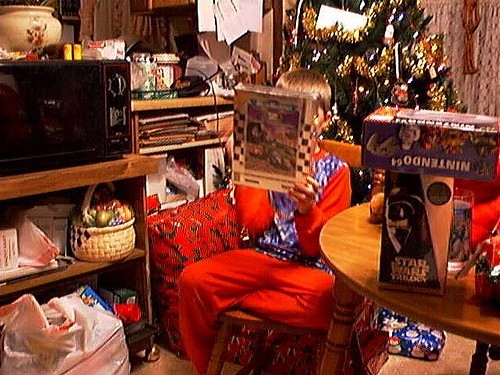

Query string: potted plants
[0,0,63,57]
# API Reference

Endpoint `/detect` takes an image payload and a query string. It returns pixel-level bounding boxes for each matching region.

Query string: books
[137,115,198,143]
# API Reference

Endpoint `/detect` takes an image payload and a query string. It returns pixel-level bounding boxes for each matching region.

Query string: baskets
[69,180,138,264]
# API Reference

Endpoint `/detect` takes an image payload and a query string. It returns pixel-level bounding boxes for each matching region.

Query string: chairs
[205,139,363,375]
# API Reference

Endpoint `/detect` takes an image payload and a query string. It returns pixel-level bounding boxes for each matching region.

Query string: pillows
[146,188,244,362]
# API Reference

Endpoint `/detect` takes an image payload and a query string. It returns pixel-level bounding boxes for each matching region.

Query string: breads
[369,193,384,223]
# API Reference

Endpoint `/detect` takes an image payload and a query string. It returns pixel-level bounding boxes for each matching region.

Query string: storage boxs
[359,105,500,183]
[376,307,446,362]
[375,171,457,298]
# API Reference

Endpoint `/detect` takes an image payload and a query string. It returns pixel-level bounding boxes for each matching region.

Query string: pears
[94,210,119,227]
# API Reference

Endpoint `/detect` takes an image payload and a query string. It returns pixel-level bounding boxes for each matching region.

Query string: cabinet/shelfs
[127,88,237,213]
[0,152,168,367]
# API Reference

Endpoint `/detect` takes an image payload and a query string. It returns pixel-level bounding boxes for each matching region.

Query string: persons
[179,68,352,375]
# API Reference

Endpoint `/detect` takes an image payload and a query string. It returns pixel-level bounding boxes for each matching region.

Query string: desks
[319,202,500,375]
